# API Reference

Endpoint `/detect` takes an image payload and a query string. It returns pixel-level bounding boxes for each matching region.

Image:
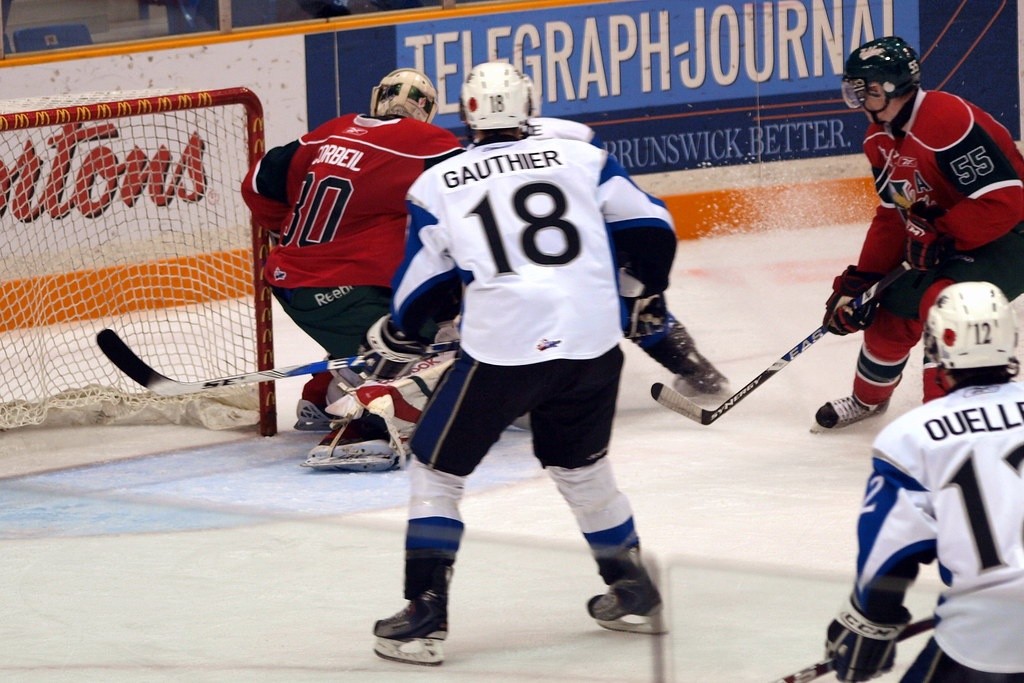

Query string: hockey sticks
[772,615,940,683]
[651,260,911,426]
[95,329,461,397]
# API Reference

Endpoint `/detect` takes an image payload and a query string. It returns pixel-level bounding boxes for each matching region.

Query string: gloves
[357,313,425,380]
[823,265,881,336]
[618,268,667,339]
[825,595,912,683]
[905,201,955,274]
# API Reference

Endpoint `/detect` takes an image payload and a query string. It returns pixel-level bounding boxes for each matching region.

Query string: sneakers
[587,560,670,634]
[673,377,735,406]
[810,395,889,434]
[373,589,449,666]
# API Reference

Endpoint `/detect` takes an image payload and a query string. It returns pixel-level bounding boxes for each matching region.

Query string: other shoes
[300,419,396,473]
[293,399,330,432]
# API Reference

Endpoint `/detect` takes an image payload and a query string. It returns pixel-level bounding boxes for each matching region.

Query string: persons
[825,281,1024,683]
[357,60,677,666]
[806,35,1024,427]
[240,63,733,471]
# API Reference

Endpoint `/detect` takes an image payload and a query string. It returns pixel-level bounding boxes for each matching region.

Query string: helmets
[460,62,528,130]
[523,75,540,118]
[924,281,1019,370]
[370,68,439,124]
[841,36,923,97]
